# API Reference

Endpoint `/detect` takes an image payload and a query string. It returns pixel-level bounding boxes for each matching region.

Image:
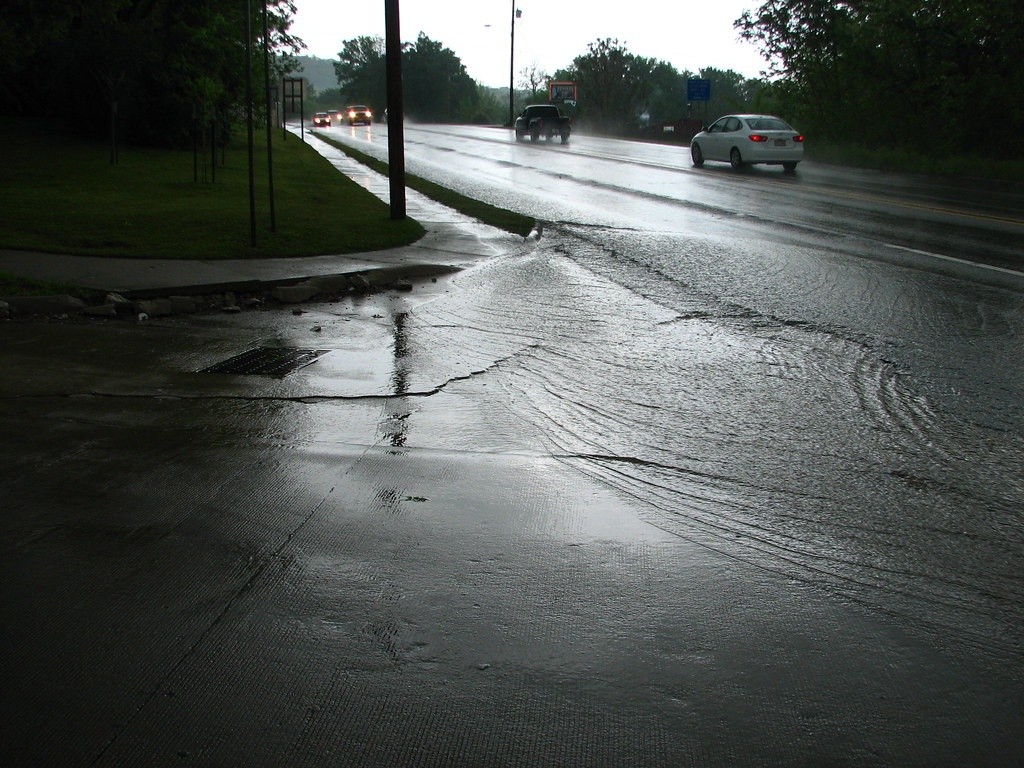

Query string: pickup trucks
[515,105,570,142]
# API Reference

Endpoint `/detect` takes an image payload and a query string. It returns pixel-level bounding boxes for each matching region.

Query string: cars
[691,113,804,173]
[343,106,372,126]
[329,110,344,125]
[313,113,332,127]
